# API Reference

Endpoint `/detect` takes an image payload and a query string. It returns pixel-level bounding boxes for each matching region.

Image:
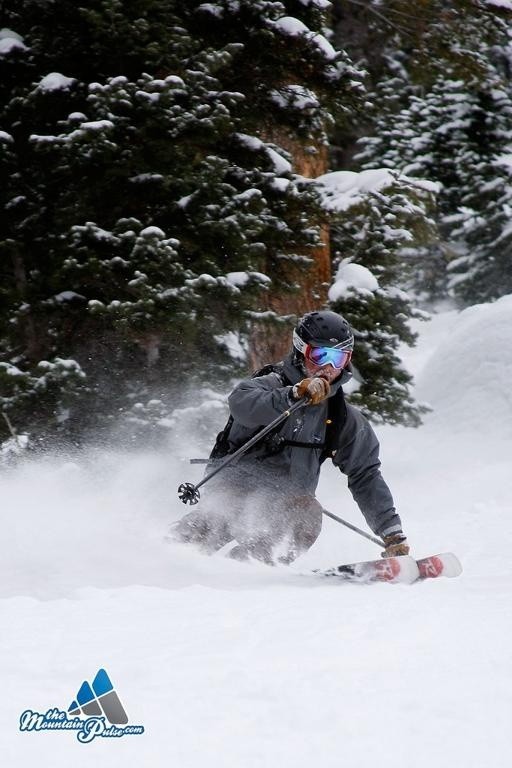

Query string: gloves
[382,533,410,557]
[292,378,330,405]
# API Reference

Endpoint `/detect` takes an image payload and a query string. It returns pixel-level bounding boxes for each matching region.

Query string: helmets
[292,311,355,353]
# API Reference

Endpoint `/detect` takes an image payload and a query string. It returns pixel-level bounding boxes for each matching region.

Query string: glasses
[306,345,351,369]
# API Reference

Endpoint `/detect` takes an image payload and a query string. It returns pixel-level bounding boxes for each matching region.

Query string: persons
[163,309,414,569]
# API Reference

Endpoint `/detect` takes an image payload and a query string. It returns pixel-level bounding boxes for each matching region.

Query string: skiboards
[317,552,462,583]
